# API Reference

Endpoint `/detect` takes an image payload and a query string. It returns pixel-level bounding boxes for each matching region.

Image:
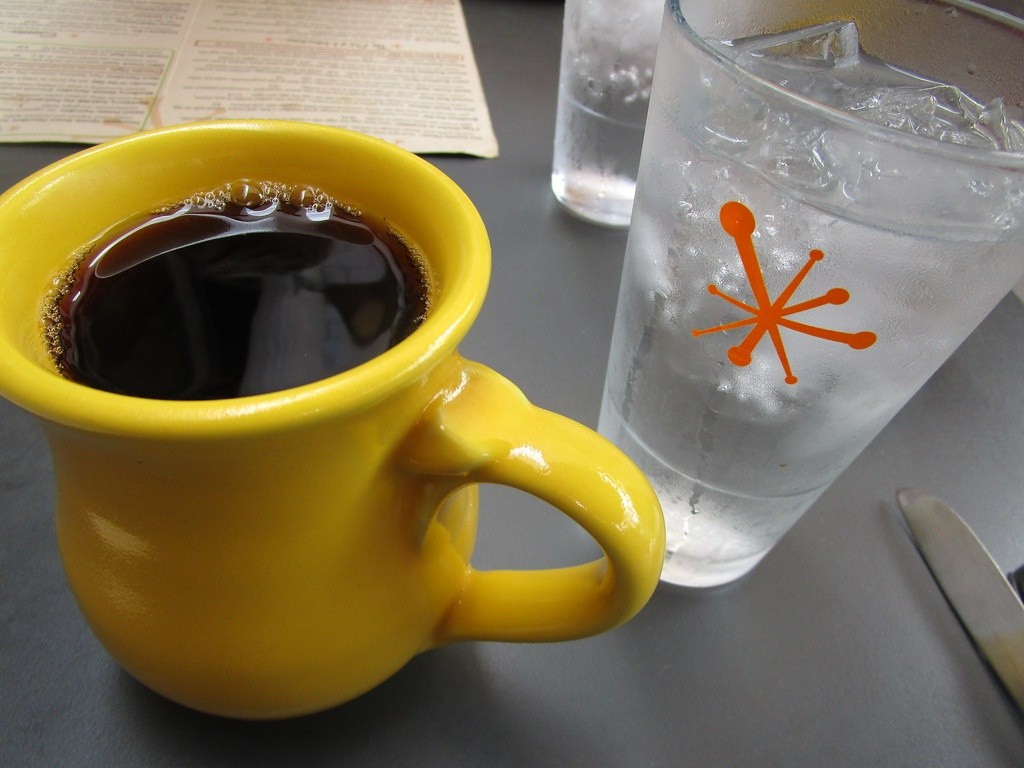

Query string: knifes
[895,487,1024,711]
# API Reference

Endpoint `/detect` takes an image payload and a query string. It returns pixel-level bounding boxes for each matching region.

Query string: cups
[549,0,664,228]
[0,120,670,723]
[596,0,1024,588]
